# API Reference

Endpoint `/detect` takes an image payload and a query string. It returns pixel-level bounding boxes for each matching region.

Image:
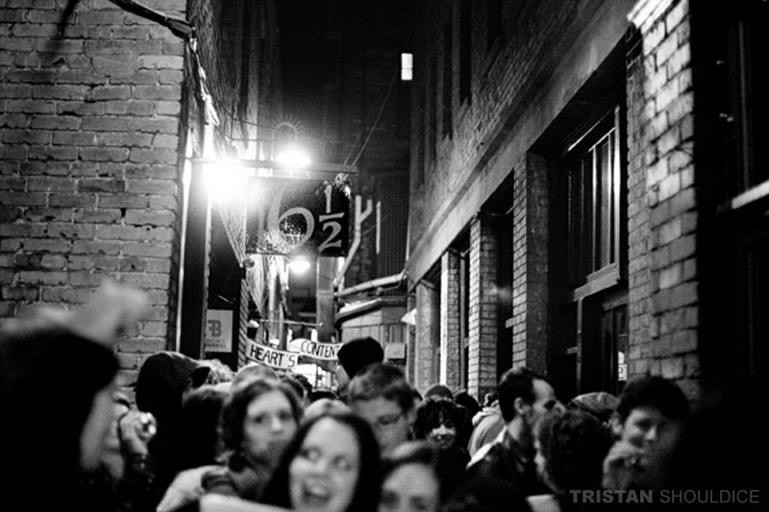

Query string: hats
[337,337,384,380]
[570,391,621,422]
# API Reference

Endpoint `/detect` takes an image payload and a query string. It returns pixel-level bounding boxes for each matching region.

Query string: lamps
[401,307,419,328]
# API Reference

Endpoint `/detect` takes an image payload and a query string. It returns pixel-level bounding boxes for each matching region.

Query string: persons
[1,276,692,512]
